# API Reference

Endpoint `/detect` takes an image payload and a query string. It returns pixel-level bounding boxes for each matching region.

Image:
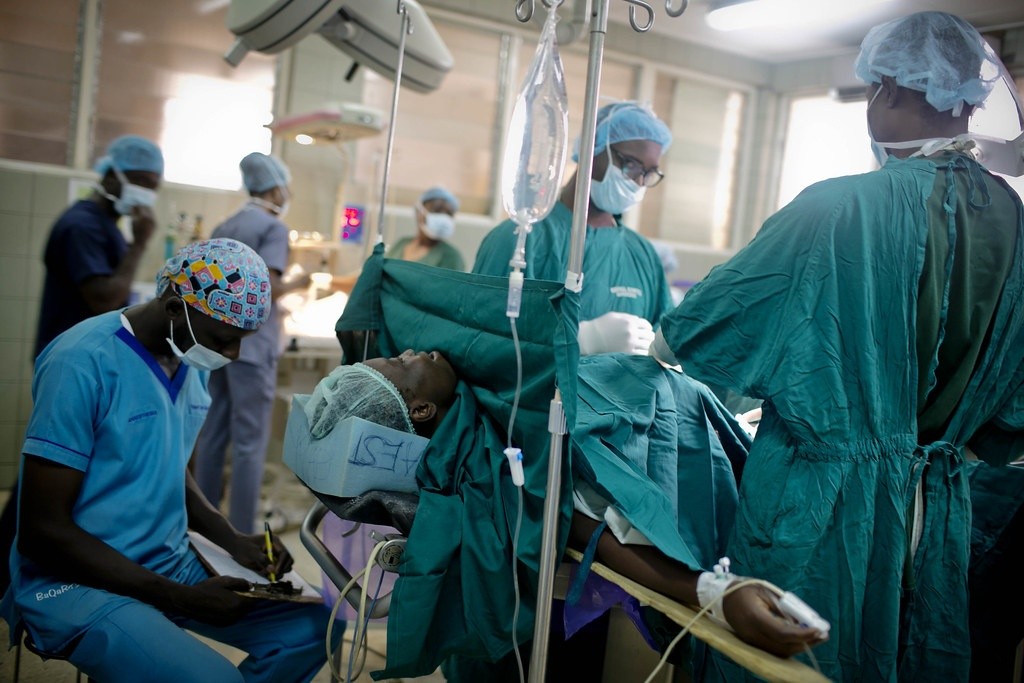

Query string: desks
[126,283,350,380]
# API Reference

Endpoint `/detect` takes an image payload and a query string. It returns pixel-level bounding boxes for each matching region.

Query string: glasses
[609,145,666,188]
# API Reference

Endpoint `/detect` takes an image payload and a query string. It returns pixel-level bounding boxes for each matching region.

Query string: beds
[281,392,624,619]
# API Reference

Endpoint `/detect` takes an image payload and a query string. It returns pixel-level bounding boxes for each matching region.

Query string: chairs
[0,475,96,683]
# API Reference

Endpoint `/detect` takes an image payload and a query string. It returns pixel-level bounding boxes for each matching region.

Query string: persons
[5,237,349,682]
[650,2,1024,683]
[309,184,468,300]
[471,102,677,361]
[190,150,312,531]
[303,347,828,664]
[31,135,166,364]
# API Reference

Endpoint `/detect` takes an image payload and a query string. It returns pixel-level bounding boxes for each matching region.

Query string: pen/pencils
[262,520,278,587]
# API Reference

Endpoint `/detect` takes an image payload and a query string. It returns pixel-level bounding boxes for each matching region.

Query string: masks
[165,299,232,371]
[590,144,647,214]
[251,193,291,217]
[865,85,968,168]
[418,204,456,242]
[91,164,157,216]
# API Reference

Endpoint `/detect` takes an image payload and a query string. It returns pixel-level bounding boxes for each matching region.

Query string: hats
[156,238,272,329]
